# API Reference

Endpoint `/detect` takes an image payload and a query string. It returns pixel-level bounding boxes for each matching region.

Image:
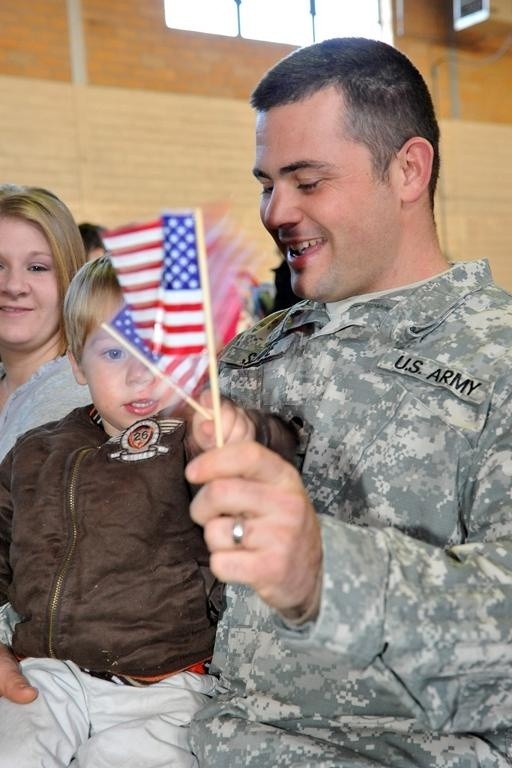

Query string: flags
[108,217,259,393]
[108,203,214,360]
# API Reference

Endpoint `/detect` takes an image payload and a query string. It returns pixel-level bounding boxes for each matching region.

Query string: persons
[171,35,510,767]
[1,255,302,765]
[1,183,100,469]
[76,222,111,264]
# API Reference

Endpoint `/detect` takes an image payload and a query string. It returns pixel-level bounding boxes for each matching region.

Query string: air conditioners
[449,0,511,47]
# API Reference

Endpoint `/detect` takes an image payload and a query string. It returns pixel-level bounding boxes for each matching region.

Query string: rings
[224,515,247,550]
